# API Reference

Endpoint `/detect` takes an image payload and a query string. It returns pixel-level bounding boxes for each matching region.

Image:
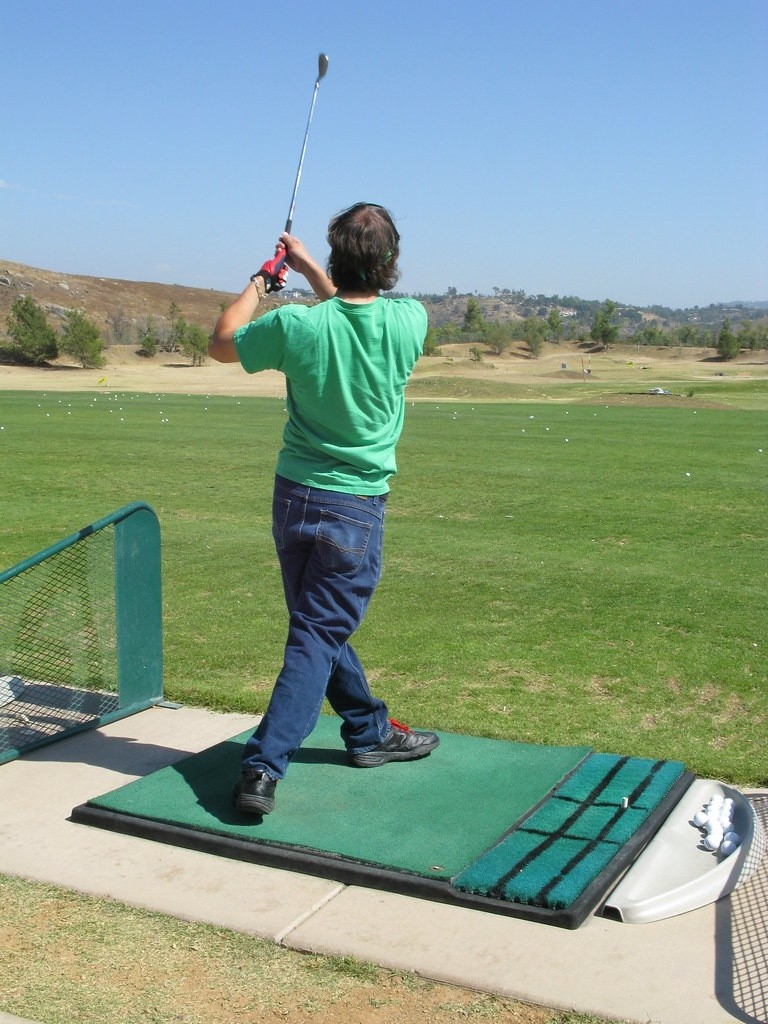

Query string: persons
[209,201,439,814]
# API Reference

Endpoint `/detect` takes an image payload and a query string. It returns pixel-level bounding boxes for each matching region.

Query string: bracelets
[252,277,268,300]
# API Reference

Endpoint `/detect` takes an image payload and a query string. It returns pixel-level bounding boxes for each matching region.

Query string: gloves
[251,249,290,293]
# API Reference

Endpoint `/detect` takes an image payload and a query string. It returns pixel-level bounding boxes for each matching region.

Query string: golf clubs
[272,51,330,293]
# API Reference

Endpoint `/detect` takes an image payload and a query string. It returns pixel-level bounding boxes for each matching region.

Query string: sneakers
[350,719,441,769]
[234,767,276,817]
